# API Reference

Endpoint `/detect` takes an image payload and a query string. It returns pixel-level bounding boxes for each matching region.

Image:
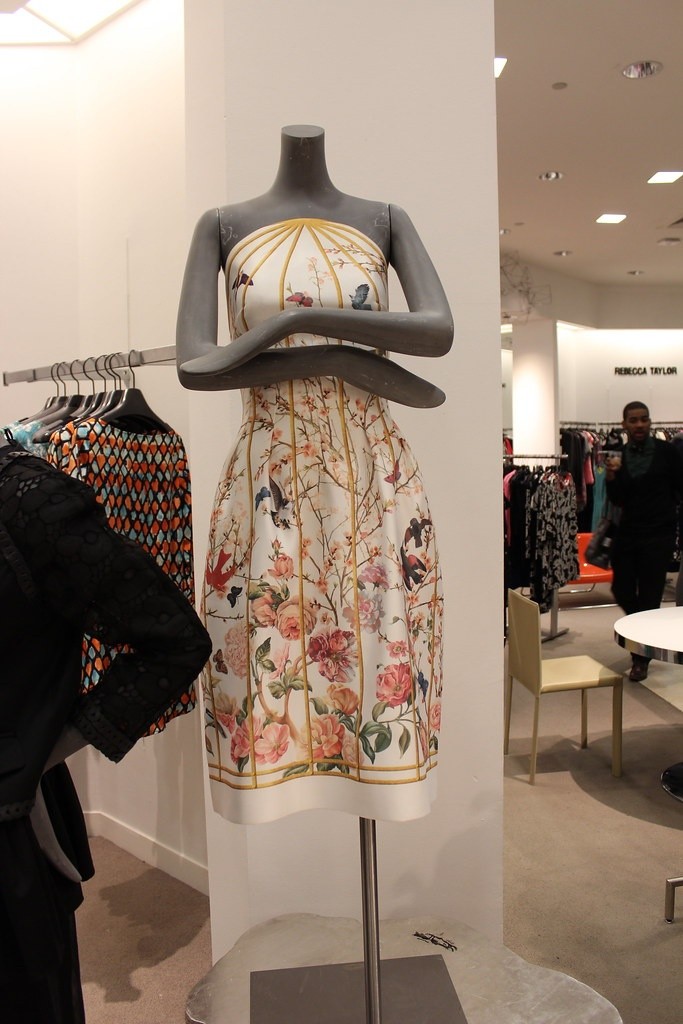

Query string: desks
[613,606,683,802]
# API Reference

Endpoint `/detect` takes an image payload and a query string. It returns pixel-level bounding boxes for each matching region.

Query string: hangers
[20,349,172,444]
[561,421,683,438]
[504,454,567,477]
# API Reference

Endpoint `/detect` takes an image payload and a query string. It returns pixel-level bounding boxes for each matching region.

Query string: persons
[601,401,682,680]
[174,126,456,821]
[0,441,211,1023]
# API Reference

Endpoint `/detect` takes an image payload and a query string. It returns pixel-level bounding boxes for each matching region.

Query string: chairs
[504,588,624,785]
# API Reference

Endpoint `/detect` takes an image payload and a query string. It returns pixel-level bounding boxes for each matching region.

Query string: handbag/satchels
[584,519,618,570]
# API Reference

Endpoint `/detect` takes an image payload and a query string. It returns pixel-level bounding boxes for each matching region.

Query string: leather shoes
[629,661,648,680]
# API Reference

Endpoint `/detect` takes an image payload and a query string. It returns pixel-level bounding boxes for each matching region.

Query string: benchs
[521,533,619,609]
